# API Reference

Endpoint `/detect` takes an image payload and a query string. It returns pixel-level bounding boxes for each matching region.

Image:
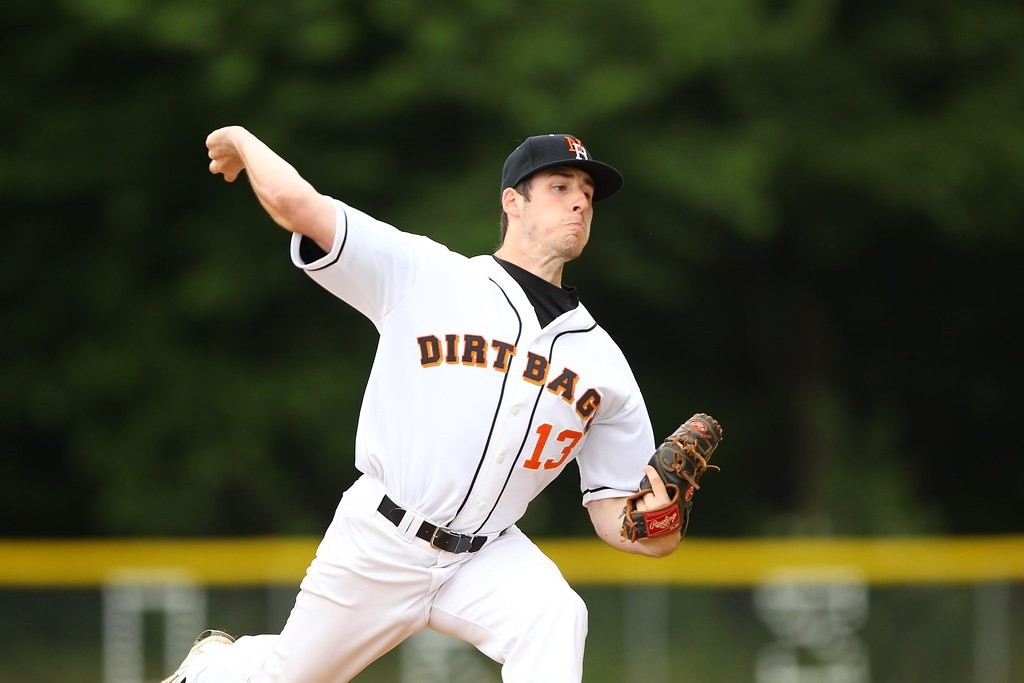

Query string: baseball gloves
[622,411,724,548]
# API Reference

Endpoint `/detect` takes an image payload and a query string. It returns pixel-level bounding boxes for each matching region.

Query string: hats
[501,133,624,203]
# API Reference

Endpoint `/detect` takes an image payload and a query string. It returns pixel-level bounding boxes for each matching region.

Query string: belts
[377,495,487,555]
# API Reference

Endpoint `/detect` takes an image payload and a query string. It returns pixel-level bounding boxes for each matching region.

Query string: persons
[157,125,680,683]
